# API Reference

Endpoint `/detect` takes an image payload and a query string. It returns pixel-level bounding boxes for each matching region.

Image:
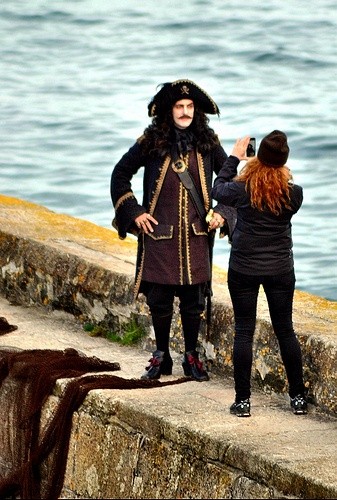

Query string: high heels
[182,351,209,381]
[141,351,174,380]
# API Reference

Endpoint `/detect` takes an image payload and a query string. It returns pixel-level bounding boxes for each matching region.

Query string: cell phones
[245,138,256,157]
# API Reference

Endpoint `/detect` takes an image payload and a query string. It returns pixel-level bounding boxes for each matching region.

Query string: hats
[257,129,289,167]
[147,79,221,116]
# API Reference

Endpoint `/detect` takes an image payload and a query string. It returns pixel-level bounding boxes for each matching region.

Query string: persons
[209,130,308,416]
[111,78,237,380]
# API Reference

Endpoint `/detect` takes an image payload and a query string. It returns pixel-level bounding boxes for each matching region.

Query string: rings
[213,215,221,227]
[135,213,151,226]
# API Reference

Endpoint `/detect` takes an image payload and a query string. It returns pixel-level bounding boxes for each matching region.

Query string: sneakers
[290,394,307,415]
[230,398,250,416]
